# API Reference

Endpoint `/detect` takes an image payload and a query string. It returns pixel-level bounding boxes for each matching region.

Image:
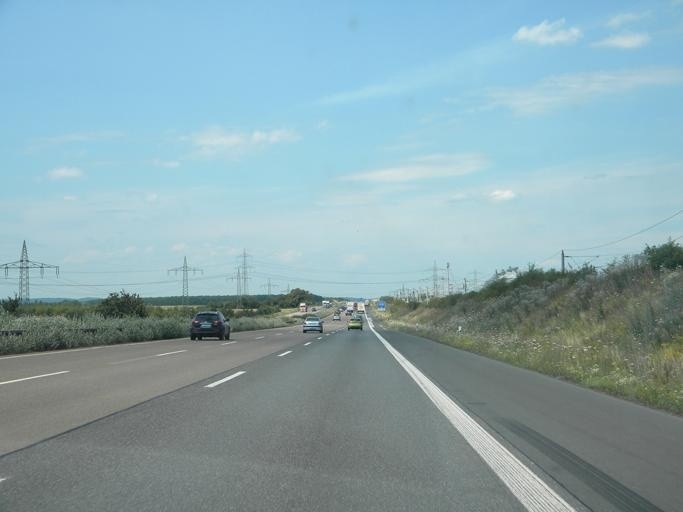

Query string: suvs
[190,311,230,341]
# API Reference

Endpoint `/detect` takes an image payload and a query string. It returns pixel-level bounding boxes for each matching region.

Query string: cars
[346,318,363,331]
[302,316,324,334]
